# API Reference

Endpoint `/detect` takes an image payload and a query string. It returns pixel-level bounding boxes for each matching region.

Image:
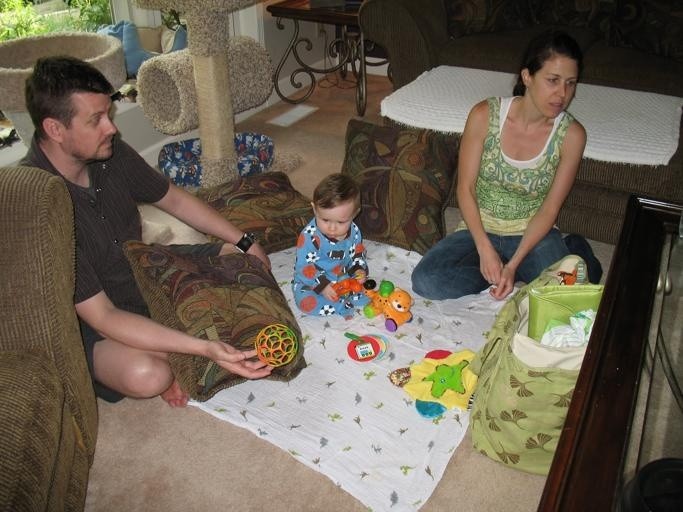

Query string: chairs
[0,162,98,511]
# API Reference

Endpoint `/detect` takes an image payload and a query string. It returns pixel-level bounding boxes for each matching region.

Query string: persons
[291,173,374,319]
[19,55,280,410]
[411,33,603,300]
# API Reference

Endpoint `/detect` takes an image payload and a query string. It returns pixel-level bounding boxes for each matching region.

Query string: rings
[490,279,496,283]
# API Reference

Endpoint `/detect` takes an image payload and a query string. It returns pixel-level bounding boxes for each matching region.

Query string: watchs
[235,231,256,253]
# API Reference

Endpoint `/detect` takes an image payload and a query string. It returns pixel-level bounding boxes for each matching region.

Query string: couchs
[357,0,682,246]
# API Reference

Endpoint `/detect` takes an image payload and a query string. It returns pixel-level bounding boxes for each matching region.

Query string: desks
[266,0,390,117]
[537,192,683,511]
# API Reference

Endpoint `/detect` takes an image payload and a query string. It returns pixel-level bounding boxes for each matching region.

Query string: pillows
[339,120,463,257]
[159,131,315,254]
[124,235,308,400]
